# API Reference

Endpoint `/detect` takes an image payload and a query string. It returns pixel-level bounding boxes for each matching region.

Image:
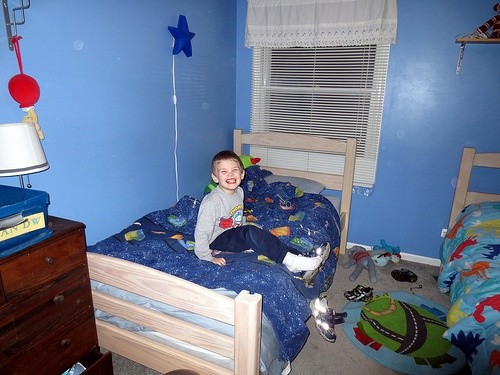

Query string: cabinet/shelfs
[0,225,114,375]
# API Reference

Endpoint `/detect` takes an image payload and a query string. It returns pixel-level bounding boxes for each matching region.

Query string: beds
[437,147,500,375]
[85,130,357,375]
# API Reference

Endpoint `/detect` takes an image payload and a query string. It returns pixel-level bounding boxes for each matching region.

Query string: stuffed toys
[344,245,377,283]
[370,240,401,266]
[477,3,500,39]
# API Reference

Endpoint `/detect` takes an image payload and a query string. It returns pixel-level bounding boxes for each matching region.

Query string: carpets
[340,290,466,375]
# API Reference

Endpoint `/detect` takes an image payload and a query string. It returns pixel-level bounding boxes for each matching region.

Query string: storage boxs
[0,186,54,258]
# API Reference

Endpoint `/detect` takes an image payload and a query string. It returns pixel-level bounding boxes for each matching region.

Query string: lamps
[0,124,49,188]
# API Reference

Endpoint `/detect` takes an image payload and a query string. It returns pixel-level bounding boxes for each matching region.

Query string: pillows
[264,175,325,195]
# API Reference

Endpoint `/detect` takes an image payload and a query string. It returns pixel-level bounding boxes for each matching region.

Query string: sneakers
[299,242,330,283]
[391,268,418,282]
[344,285,373,302]
[309,298,337,343]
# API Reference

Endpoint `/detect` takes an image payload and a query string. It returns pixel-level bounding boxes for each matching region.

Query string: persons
[194,150,331,281]
